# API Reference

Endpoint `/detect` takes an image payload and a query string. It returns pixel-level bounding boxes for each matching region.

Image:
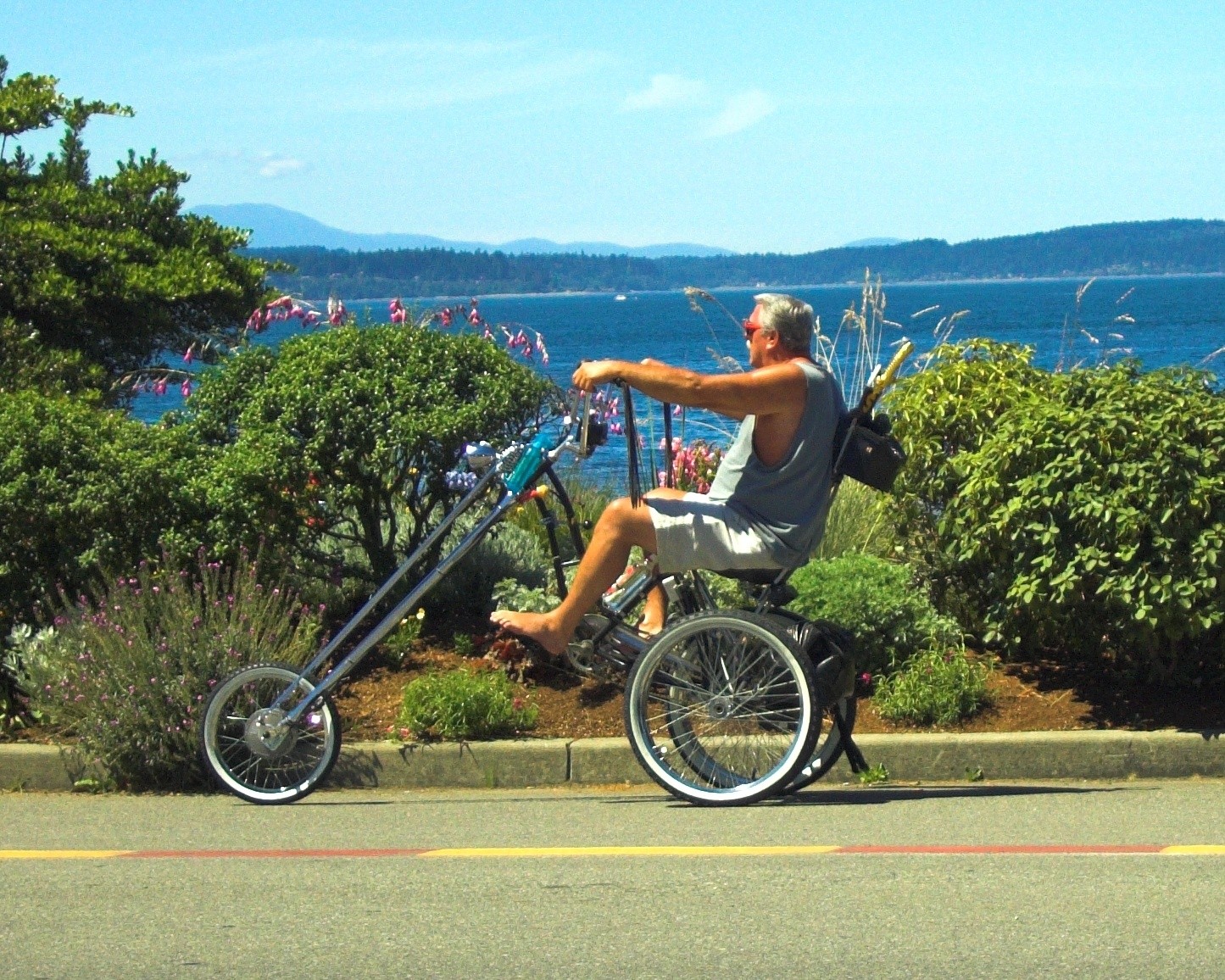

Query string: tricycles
[190,341,898,808]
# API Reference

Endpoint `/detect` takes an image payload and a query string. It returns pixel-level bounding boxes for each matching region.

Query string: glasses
[743,320,763,334]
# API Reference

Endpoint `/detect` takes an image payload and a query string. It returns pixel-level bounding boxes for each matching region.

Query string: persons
[487,291,843,660]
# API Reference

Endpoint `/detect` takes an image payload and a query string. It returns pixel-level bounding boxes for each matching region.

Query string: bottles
[504,429,552,493]
